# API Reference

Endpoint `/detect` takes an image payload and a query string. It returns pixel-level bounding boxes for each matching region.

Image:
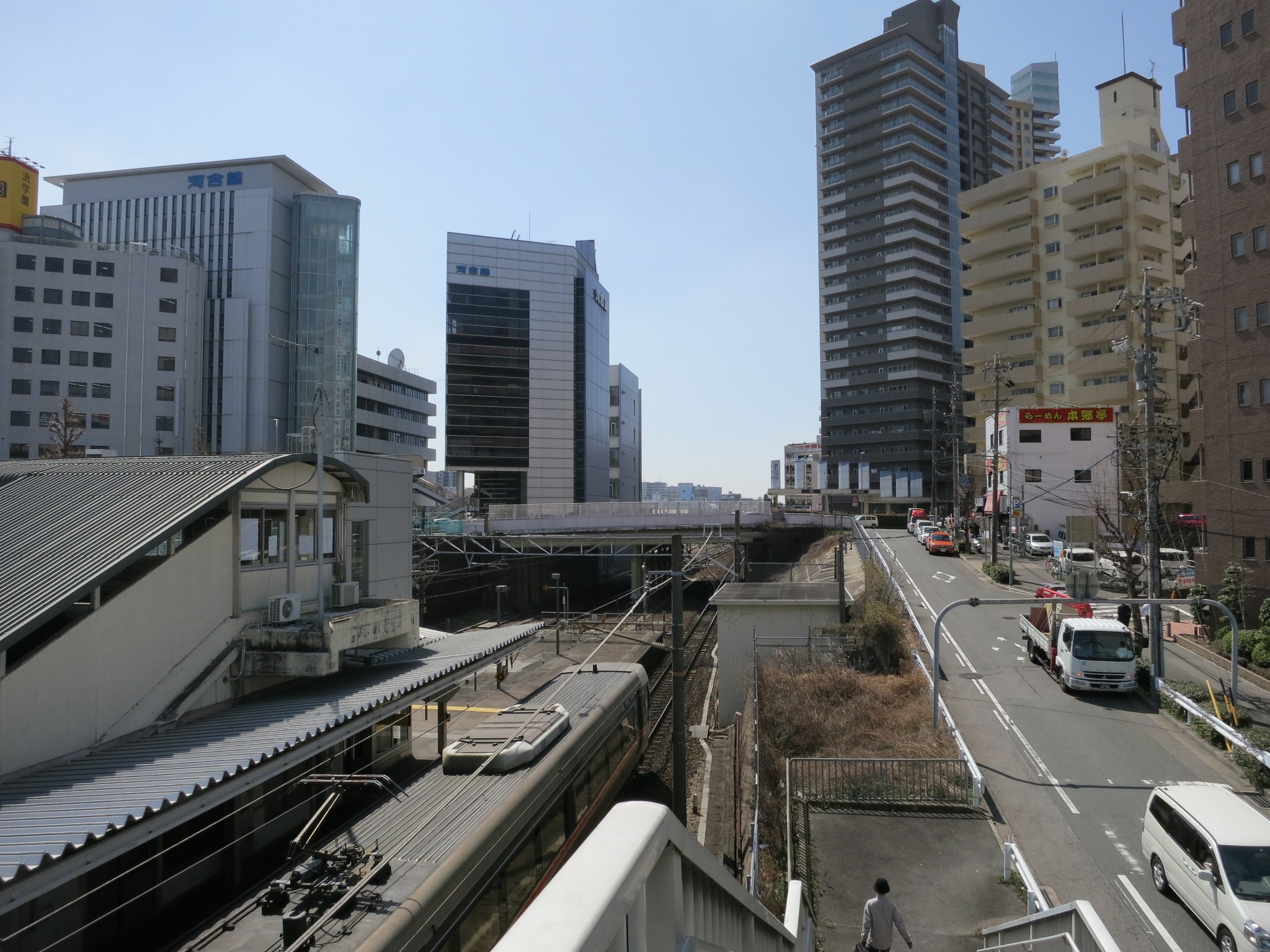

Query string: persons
[1140,604,1149,635]
[925,515,932,521]
[413,521,420,528]
[470,493,474,505]
[469,514,473,520]
[971,523,981,539]
[1117,603,1131,626]
[861,878,912,952]
[960,515,966,530]
[705,547,707,553]
[1179,565,1184,574]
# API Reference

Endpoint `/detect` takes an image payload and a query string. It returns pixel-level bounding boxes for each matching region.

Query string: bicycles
[1096,562,1124,590]
[1043,555,1061,580]
[1006,536,1024,557]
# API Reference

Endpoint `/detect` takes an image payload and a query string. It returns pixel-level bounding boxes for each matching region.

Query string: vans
[855,515,878,529]
[1141,784,1270,952]
[1063,548,1099,572]
[1141,547,1187,570]
[1098,550,1142,578]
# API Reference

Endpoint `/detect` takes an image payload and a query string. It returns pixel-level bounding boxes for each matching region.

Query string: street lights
[551,574,560,653]
[860,451,865,514]
[990,449,1014,586]
[496,584,504,626]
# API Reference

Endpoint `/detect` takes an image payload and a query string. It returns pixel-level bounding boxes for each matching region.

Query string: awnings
[973,507,982,512]
[984,490,1003,514]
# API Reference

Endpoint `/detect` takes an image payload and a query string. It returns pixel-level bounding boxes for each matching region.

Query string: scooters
[971,538,983,554]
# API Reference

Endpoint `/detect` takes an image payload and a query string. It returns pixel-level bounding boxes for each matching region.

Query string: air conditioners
[269,592,301,623]
[331,582,359,607]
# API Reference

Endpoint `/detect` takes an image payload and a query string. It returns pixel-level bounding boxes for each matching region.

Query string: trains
[181,661,653,952]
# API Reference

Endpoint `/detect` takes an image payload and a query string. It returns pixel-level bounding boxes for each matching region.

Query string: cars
[925,532,954,557]
[1025,533,1054,556]
[913,519,933,537]
[1174,513,1208,525]
[916,526,942,546]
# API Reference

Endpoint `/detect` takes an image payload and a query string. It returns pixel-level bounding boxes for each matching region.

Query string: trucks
[1019,611,1139,694]
[907,508,927,534]
